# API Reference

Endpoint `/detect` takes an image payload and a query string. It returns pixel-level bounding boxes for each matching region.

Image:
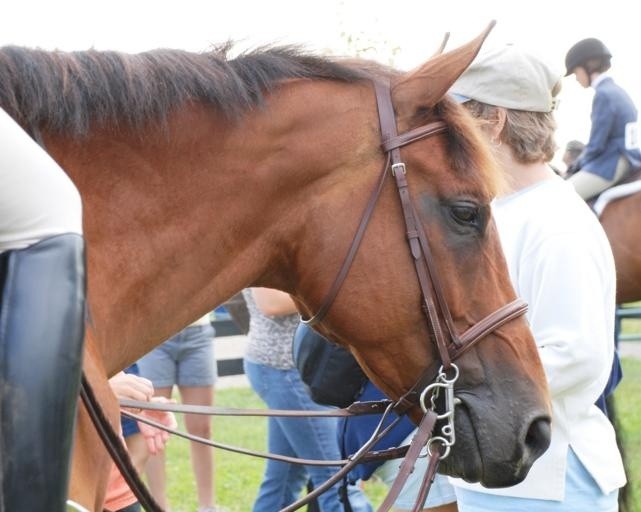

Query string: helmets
[564,38,609,75]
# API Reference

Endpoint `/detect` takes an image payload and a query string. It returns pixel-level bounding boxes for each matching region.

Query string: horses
[1,17,559,512]
[579,164,641,303]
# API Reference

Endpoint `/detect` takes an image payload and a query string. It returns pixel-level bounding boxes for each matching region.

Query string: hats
[449,42,552,113]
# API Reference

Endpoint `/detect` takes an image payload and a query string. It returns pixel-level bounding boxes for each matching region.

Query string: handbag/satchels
[292,320,367,408]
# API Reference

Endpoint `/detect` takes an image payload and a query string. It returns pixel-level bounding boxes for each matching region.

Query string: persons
[549,36,641,199]
[87,40,630,512]
